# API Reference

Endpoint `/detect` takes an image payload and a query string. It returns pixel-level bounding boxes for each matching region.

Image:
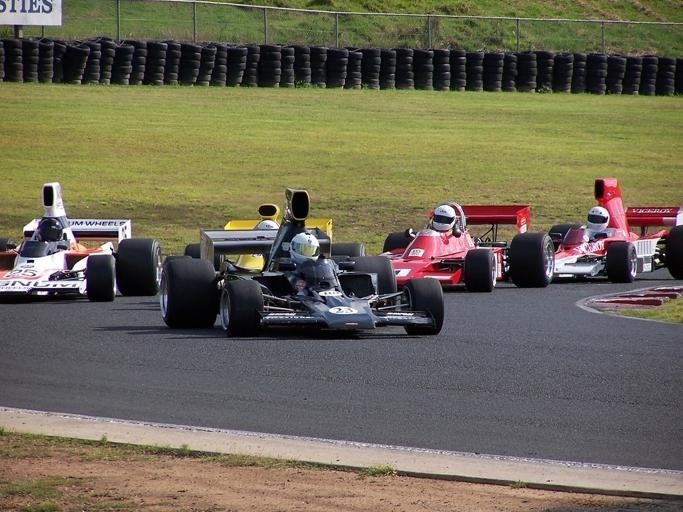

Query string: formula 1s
[378,203,555,293]
[0,182,162,303]
[160,188,445,339]
[549,180,682,283]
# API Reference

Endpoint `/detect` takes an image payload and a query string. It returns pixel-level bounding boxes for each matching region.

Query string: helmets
[289,232,321,262]
[36,217,62,241]
[587,207,610,231]
[433,205,456,231]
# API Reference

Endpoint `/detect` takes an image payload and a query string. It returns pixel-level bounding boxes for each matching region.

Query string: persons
[9,219,70,252]
[431,205,456,235]
[283,232,321,271]
[586,206,612,242]
